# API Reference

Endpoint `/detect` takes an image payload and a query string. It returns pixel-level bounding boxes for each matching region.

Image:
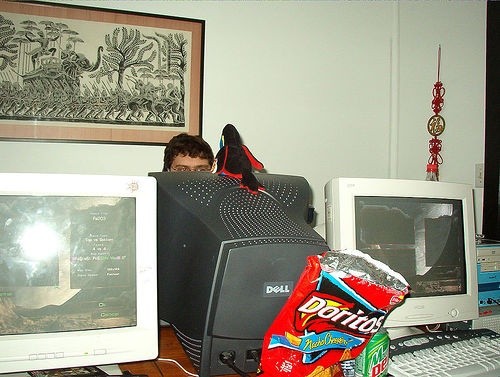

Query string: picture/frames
[0,0,206,147]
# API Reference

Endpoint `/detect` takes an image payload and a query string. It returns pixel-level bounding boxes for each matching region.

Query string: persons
[162,133,216,172]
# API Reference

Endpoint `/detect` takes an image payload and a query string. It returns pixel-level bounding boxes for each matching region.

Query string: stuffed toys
[216,123,264,194]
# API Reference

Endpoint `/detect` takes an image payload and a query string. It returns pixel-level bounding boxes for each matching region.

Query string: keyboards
[387,328,500,377]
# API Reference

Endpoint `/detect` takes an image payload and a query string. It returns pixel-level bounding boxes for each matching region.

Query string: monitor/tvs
[324,178,479,340]
[0,172,159,377]
[148,171,330,377]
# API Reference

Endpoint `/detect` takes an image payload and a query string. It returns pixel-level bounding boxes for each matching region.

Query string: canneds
[354,326,390,377]
